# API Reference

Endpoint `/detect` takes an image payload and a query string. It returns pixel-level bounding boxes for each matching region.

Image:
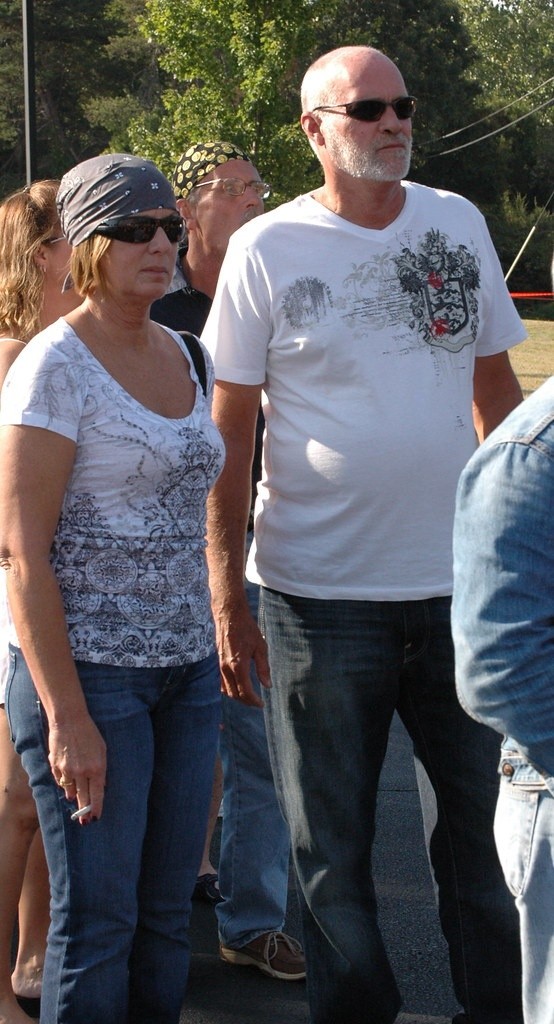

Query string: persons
[200,47,554,1024]
[0,140,307,1024]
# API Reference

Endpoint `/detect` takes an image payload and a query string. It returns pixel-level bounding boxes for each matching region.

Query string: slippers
[15,992,42,1020]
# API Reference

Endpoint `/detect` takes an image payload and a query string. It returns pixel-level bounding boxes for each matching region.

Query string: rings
[59,777,74,787]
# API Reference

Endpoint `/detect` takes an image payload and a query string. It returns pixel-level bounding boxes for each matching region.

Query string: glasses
[95,213,188,243]
[312,96,419,122]
[194,177,270,200]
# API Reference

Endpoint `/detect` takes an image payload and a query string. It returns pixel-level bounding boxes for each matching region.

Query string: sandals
[191,873,227,907]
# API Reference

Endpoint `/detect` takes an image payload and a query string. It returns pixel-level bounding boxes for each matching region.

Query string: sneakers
[220,932,307,980]
